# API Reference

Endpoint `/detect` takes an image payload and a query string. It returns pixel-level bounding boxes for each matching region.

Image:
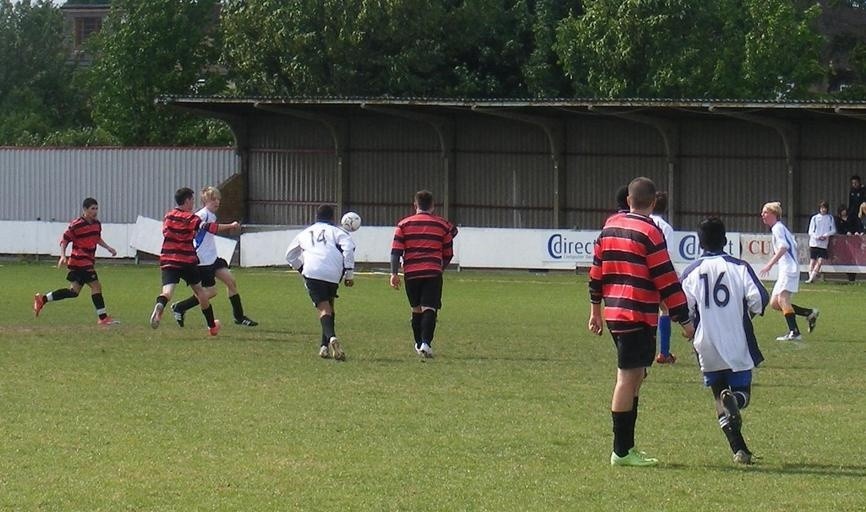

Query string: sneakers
[232,317,259,327]
[729,447,764,464]
[805,277,816,284]
[776,330,804,341]
[148,302,188,331]
[31,290,43,318]
[807,306,820,333]
[656,352,676,364]
[414,342,435,362]
[719,388,746,433]
[316,337,345,362]
[94,317,121,325]
[207,319,221,336]
[610,446,664,468]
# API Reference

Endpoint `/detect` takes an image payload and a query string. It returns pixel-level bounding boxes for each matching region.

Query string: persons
[587,175,698,469]
[758,202,819,341]
[32,199,121,327]
[835,175,866,282]
[389,190,453,357]
[805,200,836,283]
[679,214,771,466]
[151,186,258,336]
[395,211,458,239]
[615,187,677,364]
[284,203,356,361]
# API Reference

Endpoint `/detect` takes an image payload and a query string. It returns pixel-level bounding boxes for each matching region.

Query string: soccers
[341,211,361,231]
